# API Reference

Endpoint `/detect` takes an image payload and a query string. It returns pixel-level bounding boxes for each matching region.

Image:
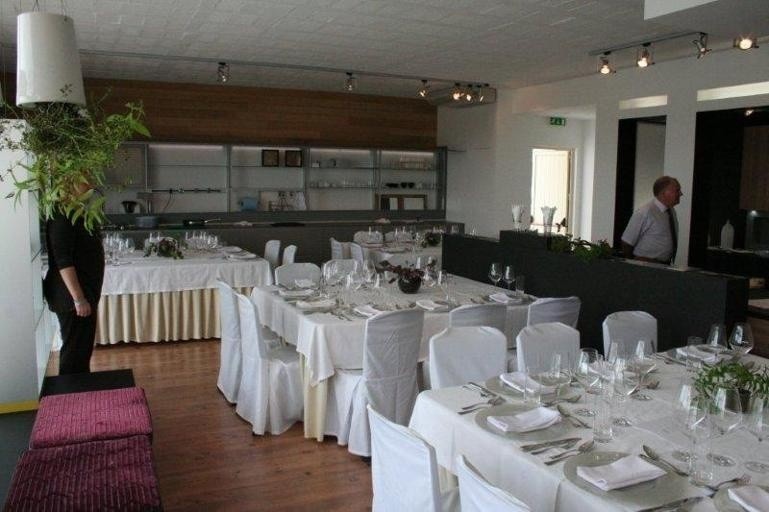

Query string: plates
[418,299,443,310]
[295,300,334,309]
[478,404,565,440]
[714,484,768,511]
[355,305,377,314]
[487,374,564,402]
[563,451,677,497]
[279,289,313,298]
[229,253,256,259]
[222,247,242,253]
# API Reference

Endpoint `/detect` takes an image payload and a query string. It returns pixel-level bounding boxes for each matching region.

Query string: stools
[2,368,161,512]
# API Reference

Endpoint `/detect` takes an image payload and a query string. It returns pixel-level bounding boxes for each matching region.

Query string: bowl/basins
[135,216,158,229]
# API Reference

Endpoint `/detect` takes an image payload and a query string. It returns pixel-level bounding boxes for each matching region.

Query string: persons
[40,173,106,379]
[619,175,683,264]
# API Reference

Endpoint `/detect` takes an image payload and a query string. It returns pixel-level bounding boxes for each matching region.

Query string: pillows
[5,386,159,512]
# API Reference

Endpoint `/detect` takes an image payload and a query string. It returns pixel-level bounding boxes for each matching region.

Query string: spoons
[552,442,595,460]
[556,404,590,429]
[642,444,687,476]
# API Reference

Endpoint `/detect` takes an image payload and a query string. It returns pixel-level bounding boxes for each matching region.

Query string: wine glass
[489,262,501,297]
[730,320,755,360]
[502,266,515,294]
[675,382,768,472]
[707,322,725,360]
[393,223,419,249]
[184,232,220,255]
[106,232,136,264]
[418,256,457,298]
[366,225,382,244]
[321,260,386,306]
[433,224,462,234]
[533,340,661,428]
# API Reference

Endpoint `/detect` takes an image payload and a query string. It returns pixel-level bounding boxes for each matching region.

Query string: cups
[593,394,611,442]
[516,276,525,296]
[686,337,702,373]
[512,201,522,225]
[541,207,555,226]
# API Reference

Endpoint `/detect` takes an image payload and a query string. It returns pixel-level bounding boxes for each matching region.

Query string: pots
[183,217,221,225]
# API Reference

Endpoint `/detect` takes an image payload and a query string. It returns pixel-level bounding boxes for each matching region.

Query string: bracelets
[71,298,89,306]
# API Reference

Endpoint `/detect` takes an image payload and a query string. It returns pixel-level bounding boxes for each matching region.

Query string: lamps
[733,32,761,51]
[78,49,489,104]
[589,31,712,76]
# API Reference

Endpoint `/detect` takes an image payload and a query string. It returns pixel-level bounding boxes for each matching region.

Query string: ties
[666,208,677,264]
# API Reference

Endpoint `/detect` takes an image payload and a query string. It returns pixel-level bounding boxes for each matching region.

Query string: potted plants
[0,12,153,236]
[689,358,769,416]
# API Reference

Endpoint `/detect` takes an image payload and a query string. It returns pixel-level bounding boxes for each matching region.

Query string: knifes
[520,439,581,449]
[633,497,701,511]
[469,382,505,403]
